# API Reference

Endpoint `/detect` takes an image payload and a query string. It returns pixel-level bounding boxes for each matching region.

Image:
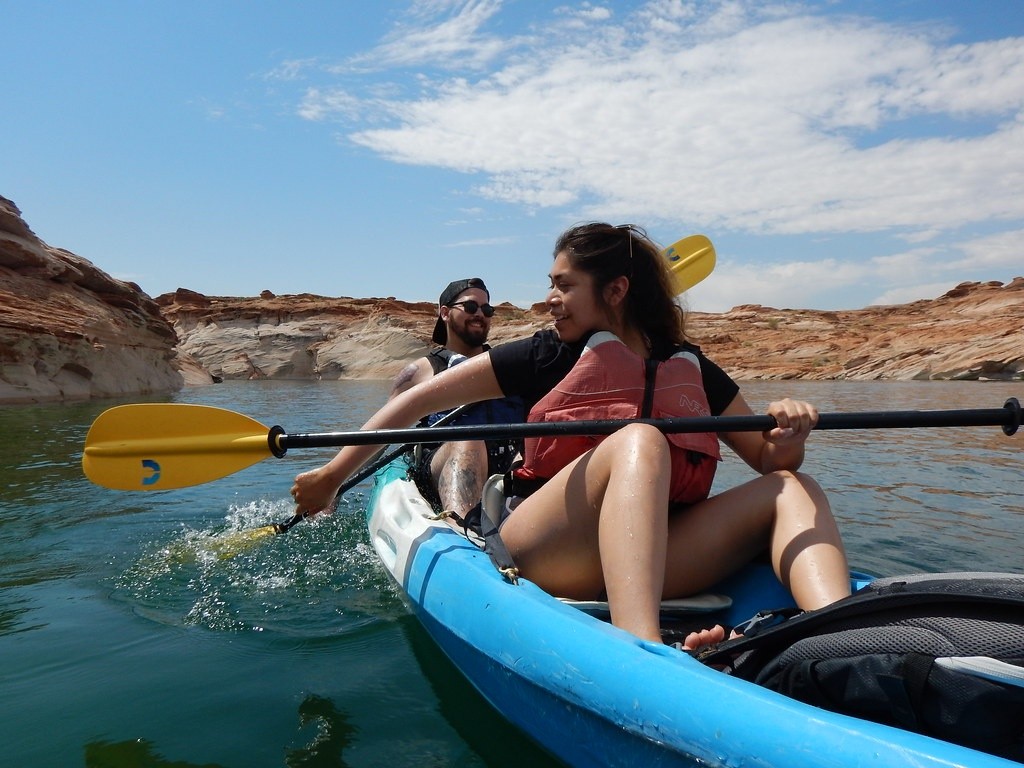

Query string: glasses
[613,224,636,258]
[451,300,496,317]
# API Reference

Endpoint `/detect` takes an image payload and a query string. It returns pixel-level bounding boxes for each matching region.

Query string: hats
[432,279,490,345]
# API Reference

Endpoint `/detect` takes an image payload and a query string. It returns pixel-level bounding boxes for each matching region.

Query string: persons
[289,222,855,654]
[319,279,530,546]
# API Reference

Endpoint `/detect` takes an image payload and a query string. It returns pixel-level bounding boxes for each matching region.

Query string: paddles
[80,394,1024,493]
[151,231,717,575]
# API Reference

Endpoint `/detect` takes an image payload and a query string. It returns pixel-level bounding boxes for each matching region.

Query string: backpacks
[723,571,1024,762]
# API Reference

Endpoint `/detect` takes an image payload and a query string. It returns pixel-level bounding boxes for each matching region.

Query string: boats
[366,454,1024,768]
